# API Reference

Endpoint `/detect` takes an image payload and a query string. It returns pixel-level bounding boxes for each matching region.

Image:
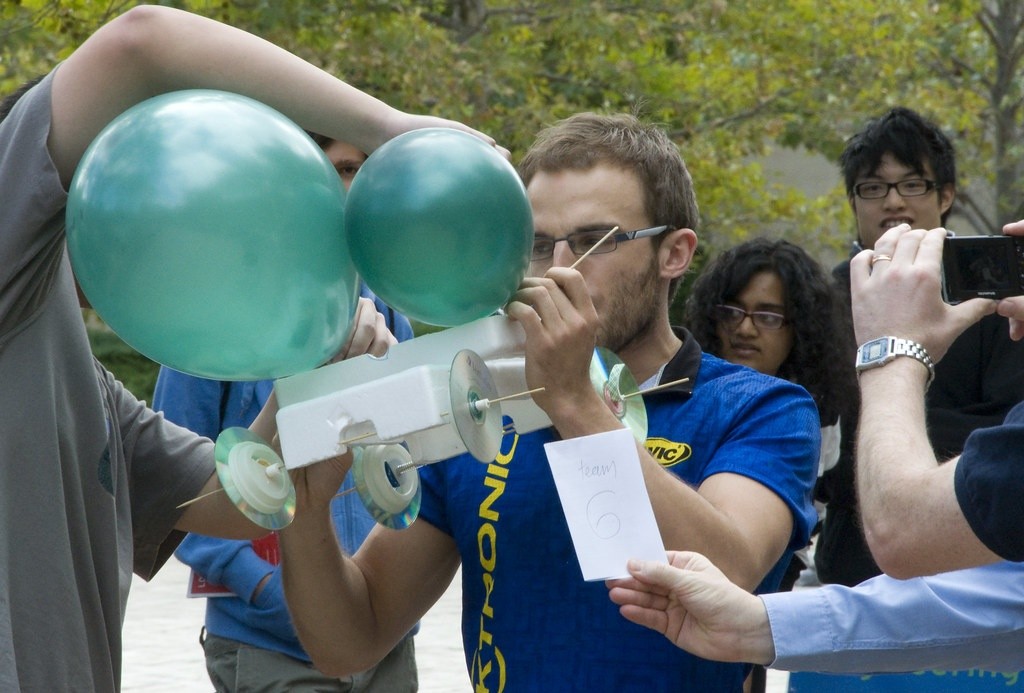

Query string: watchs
[855,336,935,396]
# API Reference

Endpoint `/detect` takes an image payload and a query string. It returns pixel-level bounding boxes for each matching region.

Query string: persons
[271,110,823,693]
[814,107,1024,588]
[850,219,1024,581]
[683,237,840,592]
[0,4,511,693]
[605,551,1024,673]
[150,136,421,693]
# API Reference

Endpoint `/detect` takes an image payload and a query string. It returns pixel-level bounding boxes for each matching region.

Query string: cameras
[942,236,1024,305]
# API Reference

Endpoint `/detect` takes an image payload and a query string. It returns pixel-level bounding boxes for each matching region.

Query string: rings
[872,254,893,264]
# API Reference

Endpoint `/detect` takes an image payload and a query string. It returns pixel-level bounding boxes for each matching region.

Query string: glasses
[531,225,674,262]
[854,180,935,200]
[718,301,789,330]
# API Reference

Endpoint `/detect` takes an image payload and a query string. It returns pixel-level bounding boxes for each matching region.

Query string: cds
[449,349,503,463]
[351,444,422,529]
[589,346,649,444]
[214,426,296,530]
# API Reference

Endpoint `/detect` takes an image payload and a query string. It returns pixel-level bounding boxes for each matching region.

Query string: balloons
[65,90,360,381]
[344,126,535,328]
